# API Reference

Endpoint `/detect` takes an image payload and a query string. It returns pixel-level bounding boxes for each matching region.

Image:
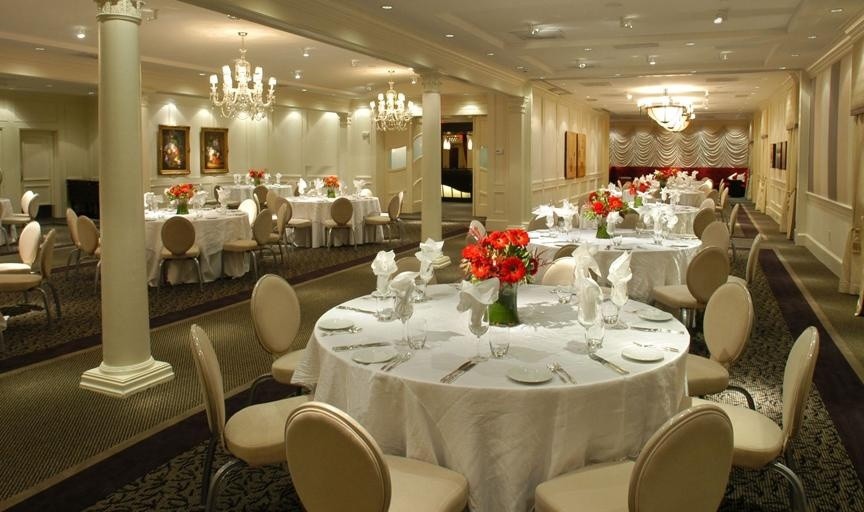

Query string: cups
[403,315,431,352]
[584,317,604,351]
[599,292,620,329]
[641,198,681,212]
[412,280,426,304]
[376,292,394,321]
[488,323,513,358]
[552,280,574,304]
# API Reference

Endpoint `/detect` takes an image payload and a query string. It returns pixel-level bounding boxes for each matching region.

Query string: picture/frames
[198,126,229,175]
[157,123,191,176]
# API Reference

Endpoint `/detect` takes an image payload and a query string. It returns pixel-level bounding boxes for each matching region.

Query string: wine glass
[542,211,671,249]
[234,173,363,200]
[145,188,230,217]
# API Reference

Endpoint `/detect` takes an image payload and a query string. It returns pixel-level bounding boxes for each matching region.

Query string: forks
[379,351,413,373]
[545,361,579,391]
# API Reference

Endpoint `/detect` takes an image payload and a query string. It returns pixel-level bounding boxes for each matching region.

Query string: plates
[507,363,554,386]
[317,317,353,329]
[621,345,665,362]
[637,307,674,323]
[351,349,398,364]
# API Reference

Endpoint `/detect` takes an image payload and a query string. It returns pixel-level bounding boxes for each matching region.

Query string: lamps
[367,75,418,135]
[207,26,278,128]
[640,100,691,135]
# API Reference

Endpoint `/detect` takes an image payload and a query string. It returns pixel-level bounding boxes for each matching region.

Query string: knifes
[333,341,390,353]
[588,349,628,378]
[439,361,476,385]
[633,325,686,337]
